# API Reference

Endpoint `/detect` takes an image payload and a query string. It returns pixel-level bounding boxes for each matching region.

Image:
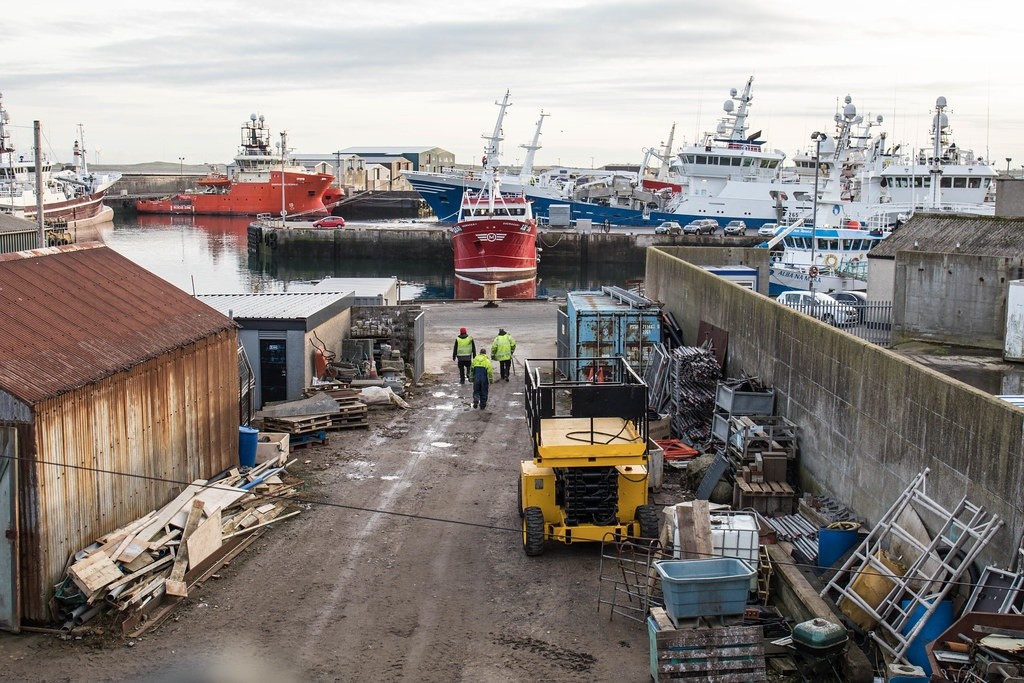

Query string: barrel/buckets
[238,424,259,470]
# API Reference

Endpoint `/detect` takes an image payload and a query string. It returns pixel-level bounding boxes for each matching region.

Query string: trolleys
[309,331,336,381]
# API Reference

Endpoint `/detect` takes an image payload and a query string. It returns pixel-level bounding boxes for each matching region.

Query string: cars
[683,220,718,235]
[723,221,747,236]
[776,291,858,327]
[757,223,779,237]
[313,216,347,229]
[655,222,682,235]
[827,292,868,313]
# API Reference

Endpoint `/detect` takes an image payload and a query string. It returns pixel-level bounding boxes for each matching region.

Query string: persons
[469,348,495,410]
[453,328,477,384]
[530,177,535,186]
[490,329,516,381]
[482,156,487,167]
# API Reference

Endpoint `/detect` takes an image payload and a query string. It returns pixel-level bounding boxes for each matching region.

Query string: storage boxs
[256,432,290,467]
[652,555,758,619]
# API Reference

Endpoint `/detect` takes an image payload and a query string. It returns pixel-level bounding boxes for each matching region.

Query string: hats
[460,328,467,335]
[480,349,487,355]
[499,328,506,332]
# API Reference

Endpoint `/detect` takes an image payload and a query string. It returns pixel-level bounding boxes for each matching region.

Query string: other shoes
[479,402,487,409]
[473,399,478,409]
[504,374,509,383]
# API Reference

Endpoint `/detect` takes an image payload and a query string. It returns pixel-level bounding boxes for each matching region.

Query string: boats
[194,163,231,186]
[398,75,1000,228]
[322,188,346,216]
[754,224,892,297]
[0,91,122,229]
[136,113,335,216]
[449,137,548,282]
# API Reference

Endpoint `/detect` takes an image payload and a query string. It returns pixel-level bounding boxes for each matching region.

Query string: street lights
[809,131,827,291]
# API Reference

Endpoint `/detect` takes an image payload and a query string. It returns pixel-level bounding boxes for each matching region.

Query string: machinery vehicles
[517,355,659,556]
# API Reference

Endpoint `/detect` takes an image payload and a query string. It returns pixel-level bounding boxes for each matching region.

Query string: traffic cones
[370,353,378,380]
[597,367,604,382]
[587,367,593,381]
